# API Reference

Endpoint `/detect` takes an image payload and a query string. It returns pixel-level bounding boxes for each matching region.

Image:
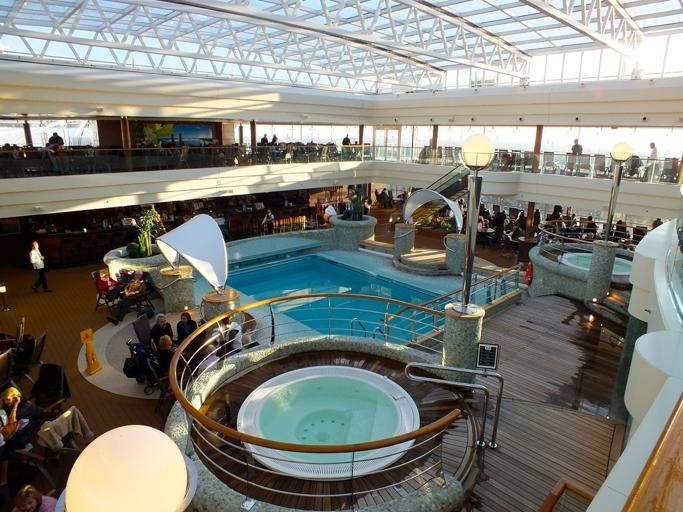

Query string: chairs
[2,140,374,178]
[2,316,97,511]
[411,145,681,184]
[3,186,347,248]
[91,267,159,323]
[372,187,649,249]
[127,313,203,416]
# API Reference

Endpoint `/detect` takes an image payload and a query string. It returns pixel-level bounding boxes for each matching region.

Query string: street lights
[601,140,633,240]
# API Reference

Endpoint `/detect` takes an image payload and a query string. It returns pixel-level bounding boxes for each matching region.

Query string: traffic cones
[81,337,105,376]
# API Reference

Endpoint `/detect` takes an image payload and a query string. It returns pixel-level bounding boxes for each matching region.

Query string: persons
[11,485,56,512]
[0,133,662,462]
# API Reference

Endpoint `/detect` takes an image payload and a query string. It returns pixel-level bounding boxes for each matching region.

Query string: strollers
[125,337,188,397]
